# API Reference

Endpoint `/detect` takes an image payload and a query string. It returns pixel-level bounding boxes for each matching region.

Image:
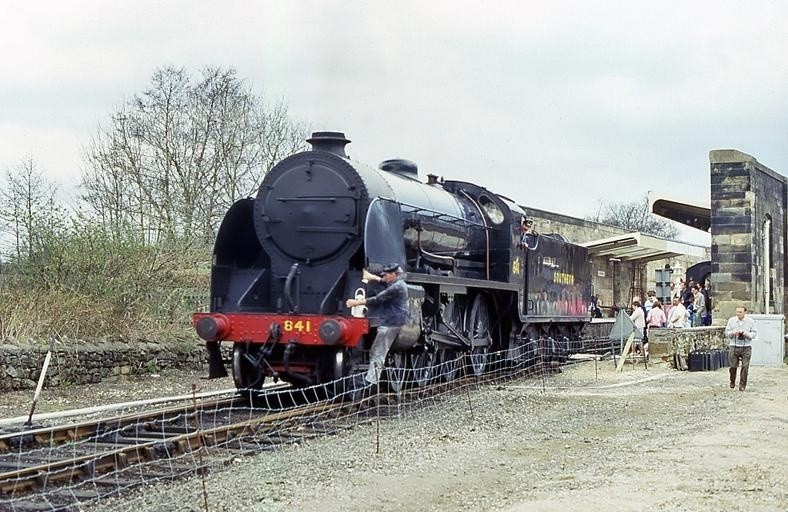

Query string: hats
[380,262,398,276]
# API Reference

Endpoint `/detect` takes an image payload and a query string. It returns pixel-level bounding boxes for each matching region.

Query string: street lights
[764,212,775,314]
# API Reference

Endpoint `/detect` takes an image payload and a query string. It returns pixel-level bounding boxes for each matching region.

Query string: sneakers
[730,381,735,388]
[739,384,745,392]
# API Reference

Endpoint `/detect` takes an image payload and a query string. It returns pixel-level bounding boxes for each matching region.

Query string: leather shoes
[356,379,373,389]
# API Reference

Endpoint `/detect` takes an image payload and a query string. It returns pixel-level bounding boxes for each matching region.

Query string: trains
[191,131,595,404]
[685,261,711,293]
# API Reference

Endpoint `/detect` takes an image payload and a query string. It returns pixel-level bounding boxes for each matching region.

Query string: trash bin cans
[688,349,730,371]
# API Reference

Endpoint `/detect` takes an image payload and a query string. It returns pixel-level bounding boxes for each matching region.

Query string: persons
[630,280,711,354]
[595,293,603,307]
[724,305,758,392]
[346,264,408,386]
